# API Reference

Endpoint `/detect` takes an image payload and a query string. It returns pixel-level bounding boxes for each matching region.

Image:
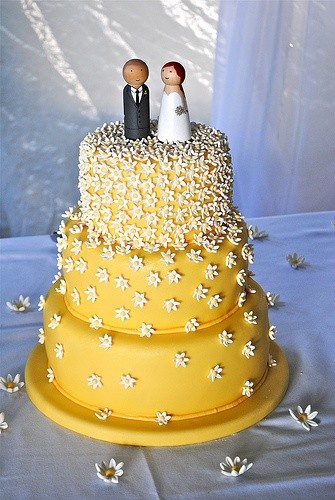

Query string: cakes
[23,59,290,448]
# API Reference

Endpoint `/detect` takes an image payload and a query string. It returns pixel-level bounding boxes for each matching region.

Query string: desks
[1,209,334,500]
